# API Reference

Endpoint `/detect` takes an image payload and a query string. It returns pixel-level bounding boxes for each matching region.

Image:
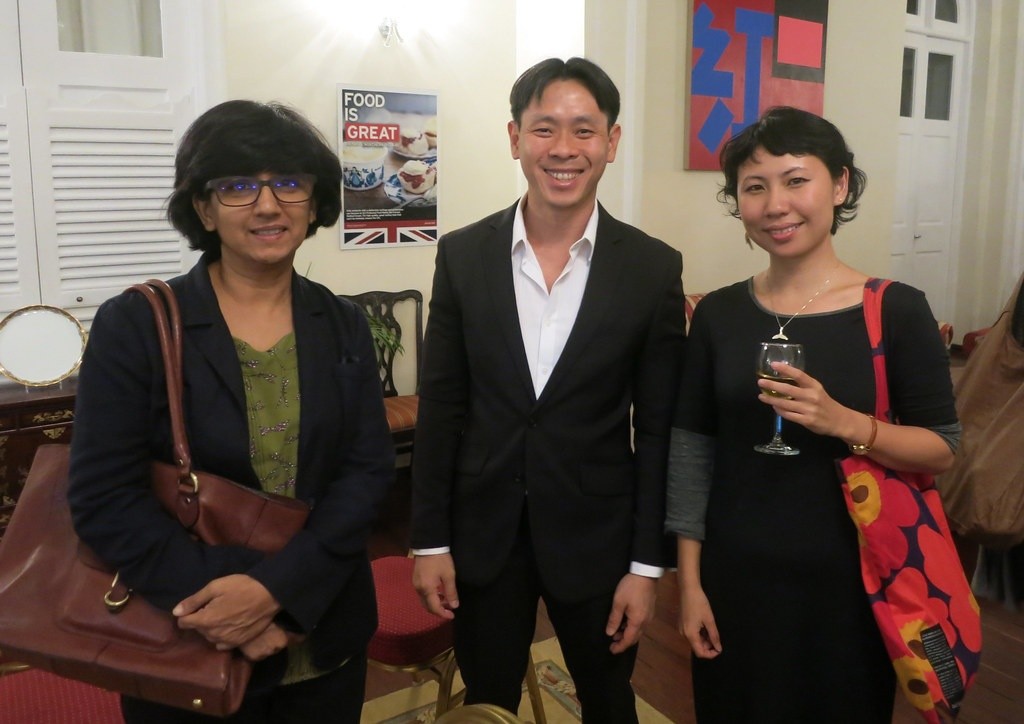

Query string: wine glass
[752,343,806,456]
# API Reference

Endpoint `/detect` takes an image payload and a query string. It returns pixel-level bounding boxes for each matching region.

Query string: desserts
[393,119,437,194]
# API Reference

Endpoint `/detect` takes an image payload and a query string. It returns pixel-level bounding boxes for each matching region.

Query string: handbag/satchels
[836,277,983,724]
[936,314,1024,548]
[0,276,309,718]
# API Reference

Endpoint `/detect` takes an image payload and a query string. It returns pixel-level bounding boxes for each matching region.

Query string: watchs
[851,414,877,454]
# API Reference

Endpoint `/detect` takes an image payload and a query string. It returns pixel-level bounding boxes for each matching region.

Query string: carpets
[357,634,676,724]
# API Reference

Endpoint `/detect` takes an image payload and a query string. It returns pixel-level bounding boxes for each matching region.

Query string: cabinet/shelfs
[0,373,77,543]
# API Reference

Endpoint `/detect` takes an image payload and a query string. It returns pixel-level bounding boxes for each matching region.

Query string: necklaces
[766,261,844,340]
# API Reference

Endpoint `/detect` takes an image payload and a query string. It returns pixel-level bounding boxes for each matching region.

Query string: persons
[406,58,686,724]
[665,105,964,724]
[66,101,397,724]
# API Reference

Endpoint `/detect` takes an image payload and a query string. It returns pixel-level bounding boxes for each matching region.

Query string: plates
[383,172,437,208]
[390,144,437,162]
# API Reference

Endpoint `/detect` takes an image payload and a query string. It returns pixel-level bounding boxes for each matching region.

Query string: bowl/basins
[344,144,387,190]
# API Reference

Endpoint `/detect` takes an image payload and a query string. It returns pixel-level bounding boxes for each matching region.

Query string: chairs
[364,538,547,724]
[338,288,433,480]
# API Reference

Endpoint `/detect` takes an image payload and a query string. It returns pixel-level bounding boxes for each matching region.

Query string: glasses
[203,173,317,207]
